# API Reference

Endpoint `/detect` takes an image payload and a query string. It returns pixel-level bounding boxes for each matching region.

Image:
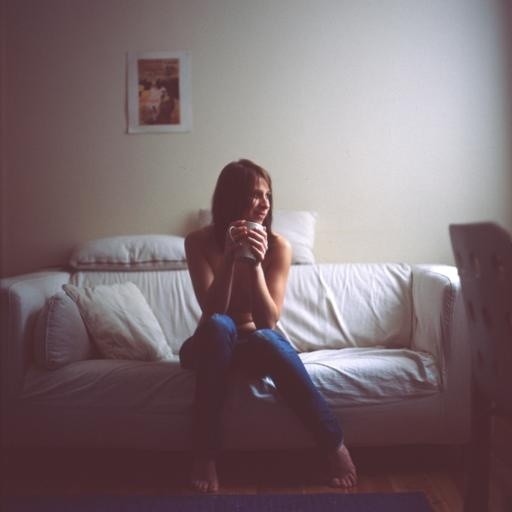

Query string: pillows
[62,281,174,361]
[33,291,98,370]
[67,235,187,270]
[199,207,318,264]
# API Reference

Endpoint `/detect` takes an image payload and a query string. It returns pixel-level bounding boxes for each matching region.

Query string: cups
[227,220,264,264]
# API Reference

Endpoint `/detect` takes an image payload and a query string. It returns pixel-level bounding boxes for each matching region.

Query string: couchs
[0,262,472,475]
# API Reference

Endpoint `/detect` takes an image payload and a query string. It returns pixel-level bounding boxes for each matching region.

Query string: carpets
[0,491,435,512]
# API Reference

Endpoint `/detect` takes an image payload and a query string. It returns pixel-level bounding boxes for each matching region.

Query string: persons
[174,158,357,493]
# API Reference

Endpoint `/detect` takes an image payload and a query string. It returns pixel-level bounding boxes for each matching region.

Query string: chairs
[449,222,512,512]
